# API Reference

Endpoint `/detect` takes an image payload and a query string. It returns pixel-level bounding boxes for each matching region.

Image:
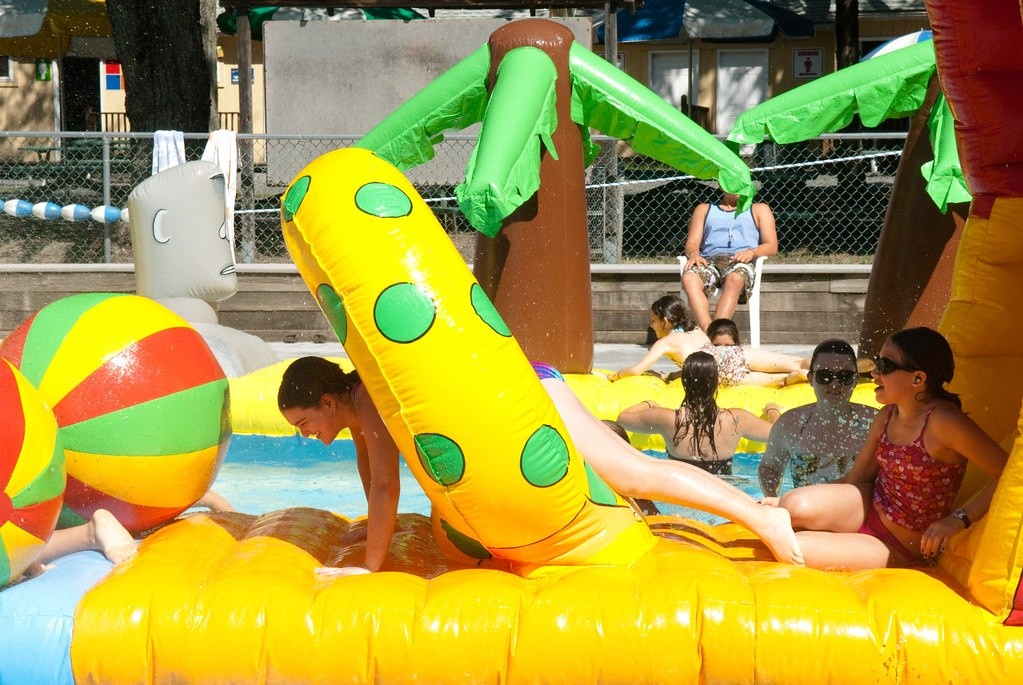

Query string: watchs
[950,507,972,529]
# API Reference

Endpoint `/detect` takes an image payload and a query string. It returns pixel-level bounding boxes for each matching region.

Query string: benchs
[11,160,131,197]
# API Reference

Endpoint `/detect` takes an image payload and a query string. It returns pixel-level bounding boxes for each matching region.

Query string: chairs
[677,255,769,345]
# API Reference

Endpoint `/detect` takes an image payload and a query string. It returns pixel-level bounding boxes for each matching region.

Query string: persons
[758,339,880,499]
[277,358,804,577]
[616,352,777,478]
[680,175,779,335]
[21,488,238,580]
[606,295,876,388]
[754,327,1010,571]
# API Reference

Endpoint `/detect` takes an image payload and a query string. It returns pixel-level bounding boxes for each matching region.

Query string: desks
[75,140,131,161]
[18,145,118,176]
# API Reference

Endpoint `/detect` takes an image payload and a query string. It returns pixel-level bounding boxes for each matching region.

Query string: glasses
[811,369,858,385]
[872,354,916,374]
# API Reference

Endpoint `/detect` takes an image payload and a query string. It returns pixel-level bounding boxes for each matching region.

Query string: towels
[152,130,241,271]
